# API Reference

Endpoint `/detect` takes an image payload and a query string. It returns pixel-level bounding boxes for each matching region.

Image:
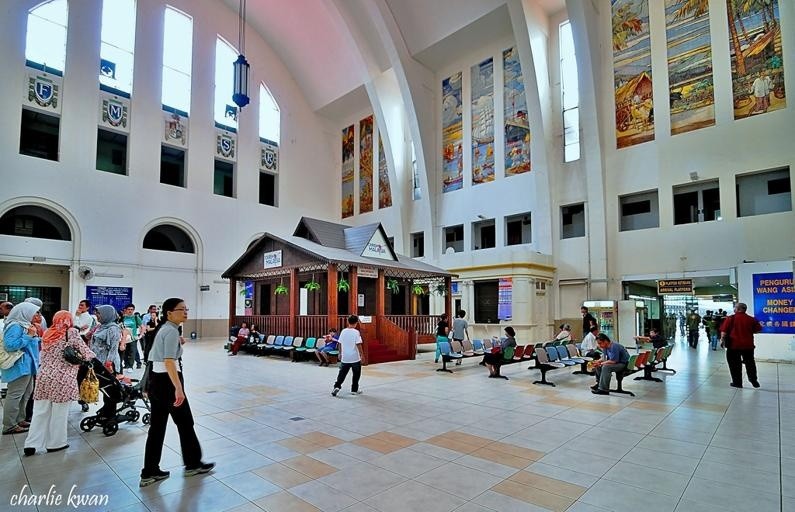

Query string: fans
[78,266,94,280]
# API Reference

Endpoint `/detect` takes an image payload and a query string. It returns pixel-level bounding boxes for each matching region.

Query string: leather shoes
[3,417,32,434]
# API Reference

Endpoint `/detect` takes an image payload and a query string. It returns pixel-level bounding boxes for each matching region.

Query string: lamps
[233,0,250,109]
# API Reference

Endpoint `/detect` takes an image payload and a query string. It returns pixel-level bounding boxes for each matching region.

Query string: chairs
[436,337,676,396]
[226,332,340,356]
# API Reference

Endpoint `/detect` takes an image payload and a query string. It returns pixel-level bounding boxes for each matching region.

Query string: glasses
[170,308,189,312]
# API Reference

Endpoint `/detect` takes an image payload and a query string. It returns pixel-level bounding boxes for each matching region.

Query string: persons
[590,333,630,395]
[484,325,516,377]
[751,67,773,113]
[140,298,215,487]
[555,307,728,360]
[227,322,260,357]
[1,297,161,456]
[314,328,339,366]
[452,310,469,365]
[719,303,762,388]
[331,314,365,396]
[435,314,452,363]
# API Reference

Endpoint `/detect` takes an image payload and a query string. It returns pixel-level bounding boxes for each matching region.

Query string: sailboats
[473,144,483,183]
[473,104,494,144]
[442,141,458,163]
[505,84,529,141]
[481,143,495,170]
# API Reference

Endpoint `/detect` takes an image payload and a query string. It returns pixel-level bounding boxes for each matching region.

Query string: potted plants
[274,287,288,296]
[304,282,320,291]
[338,278,350,292]
[386,277,399,295]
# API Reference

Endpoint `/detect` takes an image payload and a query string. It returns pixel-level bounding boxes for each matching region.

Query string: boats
[443,174,463,186]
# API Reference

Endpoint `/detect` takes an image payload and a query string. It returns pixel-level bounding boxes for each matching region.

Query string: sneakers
[331,387,339,396]
[123,363,141,373]
[81,404,89,411]
[749,377,760,387]
[23,448,36,456]
[47,444,69,452]
[325,360,330,366]
[184,462,213,477]
[351,389,362,395]
[140,470,169,487]
[590,384,609,395]
[319,360,324,366]
[731,383,742,387]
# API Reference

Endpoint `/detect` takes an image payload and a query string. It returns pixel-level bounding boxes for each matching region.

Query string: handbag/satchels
[63,345,83,365]
[120,327,132,343]
[0,333,24,370]
[448,331,454,339]
[491,347,501,354]
[80,379,100,403]
[136,328,146,339]
[142,361,184,392]
[725,334,730,347]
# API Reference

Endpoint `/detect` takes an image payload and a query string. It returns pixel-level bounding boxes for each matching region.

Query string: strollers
[80,356,151,436]
